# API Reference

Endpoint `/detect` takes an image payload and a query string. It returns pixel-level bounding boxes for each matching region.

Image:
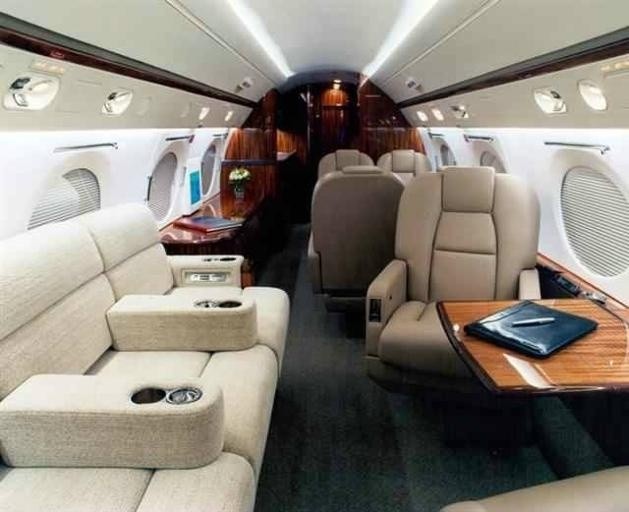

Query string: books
[174,213,245,234]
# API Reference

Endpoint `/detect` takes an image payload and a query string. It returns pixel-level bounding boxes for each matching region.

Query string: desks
[160,182,265,258]
[433,295,629,397]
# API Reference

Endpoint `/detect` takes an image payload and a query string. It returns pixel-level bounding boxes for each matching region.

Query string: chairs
[437,463,627,512]
[363,166,543,405]
[377,148,433,185]
[318,148,374,176]
[307,166,404,312]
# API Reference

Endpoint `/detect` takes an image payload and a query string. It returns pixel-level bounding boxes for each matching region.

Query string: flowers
[227,167,250,190]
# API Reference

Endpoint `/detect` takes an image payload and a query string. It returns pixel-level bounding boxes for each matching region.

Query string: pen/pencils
[512,317,555,327]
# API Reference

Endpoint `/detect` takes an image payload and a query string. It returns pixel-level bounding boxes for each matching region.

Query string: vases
[234,183,245,199]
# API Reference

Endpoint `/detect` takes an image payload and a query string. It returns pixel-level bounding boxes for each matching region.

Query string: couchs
[0,202,290,509]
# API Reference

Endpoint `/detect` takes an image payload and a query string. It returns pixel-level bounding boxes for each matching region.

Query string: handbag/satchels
[463,300,597,359]
